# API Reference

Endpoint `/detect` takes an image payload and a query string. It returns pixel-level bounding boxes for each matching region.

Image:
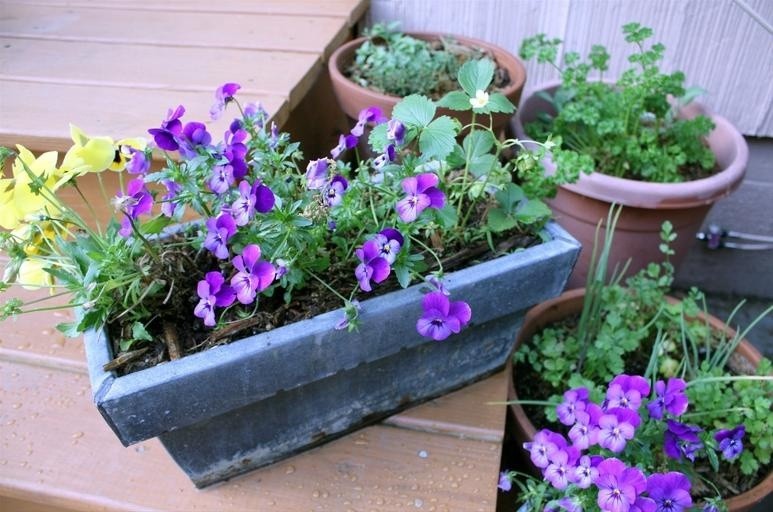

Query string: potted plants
[329,27,527,172]
[0,58,562,342]
[505,22,749,289]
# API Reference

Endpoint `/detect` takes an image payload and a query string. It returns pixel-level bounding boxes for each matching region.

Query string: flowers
[498,374,745,512]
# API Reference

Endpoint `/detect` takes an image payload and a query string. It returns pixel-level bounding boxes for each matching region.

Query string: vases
[508,286,773,510]
[76,152,584,489]
